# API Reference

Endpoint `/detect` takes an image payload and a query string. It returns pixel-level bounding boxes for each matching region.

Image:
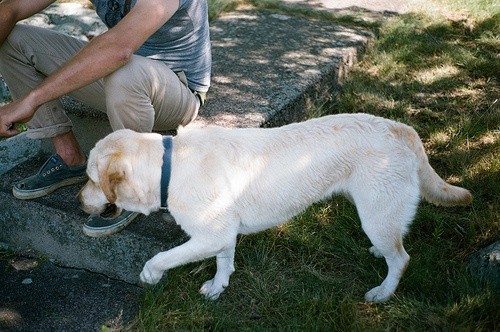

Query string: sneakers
[83,201,141,237]
[11,153,91,200]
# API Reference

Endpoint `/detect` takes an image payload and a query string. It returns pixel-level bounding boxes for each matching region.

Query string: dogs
[72,112,473,304]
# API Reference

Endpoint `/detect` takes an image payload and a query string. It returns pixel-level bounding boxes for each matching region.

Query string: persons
[0,0,212,237]
